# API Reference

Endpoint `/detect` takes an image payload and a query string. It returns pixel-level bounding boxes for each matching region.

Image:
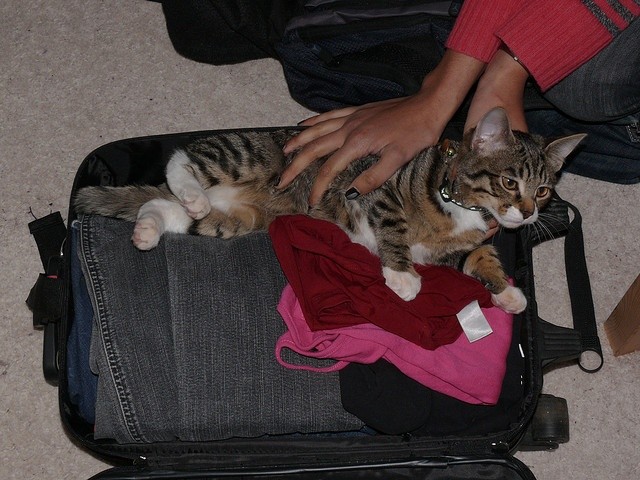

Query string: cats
[73,107,588,314]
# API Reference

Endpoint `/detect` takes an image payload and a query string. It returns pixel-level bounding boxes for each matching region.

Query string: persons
[274,2,639,210]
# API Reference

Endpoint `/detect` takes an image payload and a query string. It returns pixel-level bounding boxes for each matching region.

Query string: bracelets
[499,40,535,80]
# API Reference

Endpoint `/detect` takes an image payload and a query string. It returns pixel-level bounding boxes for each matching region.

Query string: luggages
[25,117,604,479]
